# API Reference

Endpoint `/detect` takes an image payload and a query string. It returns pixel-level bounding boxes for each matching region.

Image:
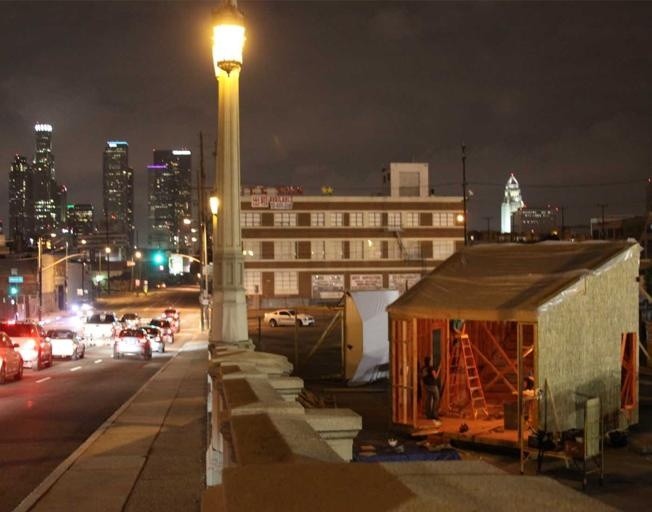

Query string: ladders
[437,334,488,421]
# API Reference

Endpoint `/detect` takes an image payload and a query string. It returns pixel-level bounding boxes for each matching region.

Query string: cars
[158,282,167,289]
[70,298,181,361]
[1,331,24,385]
[46,329,86,361]
[263,308,315,328]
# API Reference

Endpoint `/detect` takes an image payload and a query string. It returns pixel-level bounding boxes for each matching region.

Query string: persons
[448,318,465,369]
[512,376,535,424]
[420,355,443,419]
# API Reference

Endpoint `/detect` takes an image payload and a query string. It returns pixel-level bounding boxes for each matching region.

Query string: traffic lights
[11,285,17,299]
[155,251,164,266]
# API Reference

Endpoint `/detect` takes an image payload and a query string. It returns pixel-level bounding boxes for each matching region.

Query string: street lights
[183,217,210,320]
[208,0,249,343]
[36,232,56,322]
[81,238,87,304]
[210,190,218,334]
[106,246,111,297]
[130,249,141,297]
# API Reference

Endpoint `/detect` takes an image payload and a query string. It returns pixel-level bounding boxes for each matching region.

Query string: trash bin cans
[503,400,519,430]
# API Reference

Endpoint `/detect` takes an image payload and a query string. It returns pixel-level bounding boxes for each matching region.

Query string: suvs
[1,319,52,371]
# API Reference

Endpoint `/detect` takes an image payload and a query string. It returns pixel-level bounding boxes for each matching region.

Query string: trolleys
[534,394,604,491]
